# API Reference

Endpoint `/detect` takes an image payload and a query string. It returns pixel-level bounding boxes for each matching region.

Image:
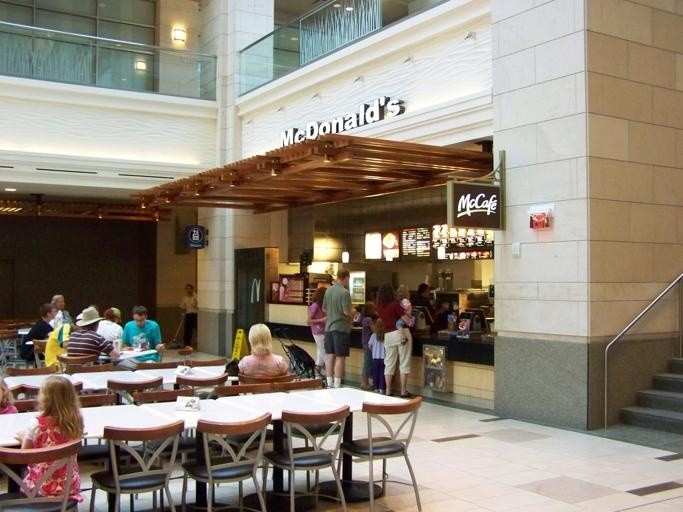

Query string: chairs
[4,321,325,404]
[90,417,186,511]
[344,393,422,512]
[2,440,83,511]
[263,403,348,510]
[184,410,274,512]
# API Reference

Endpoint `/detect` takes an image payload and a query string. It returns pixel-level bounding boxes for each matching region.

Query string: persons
[179,285,197,346]
[238,322,287,384]
[20,295,165,374]
[14,375,83,503]
[307,269,445,398]
[0,377,22,498]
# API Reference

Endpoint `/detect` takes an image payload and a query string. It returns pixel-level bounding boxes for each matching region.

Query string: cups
[113,337,120,353]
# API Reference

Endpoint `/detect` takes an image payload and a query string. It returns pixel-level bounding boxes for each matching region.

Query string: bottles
[446,311,454,332]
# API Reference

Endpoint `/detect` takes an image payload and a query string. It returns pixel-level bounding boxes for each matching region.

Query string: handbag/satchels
[224,358,239,377]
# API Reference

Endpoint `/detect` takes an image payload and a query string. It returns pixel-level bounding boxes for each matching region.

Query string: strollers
[272,326,326,389]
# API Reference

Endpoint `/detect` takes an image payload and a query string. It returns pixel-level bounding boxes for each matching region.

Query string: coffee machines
[435,291,467,330]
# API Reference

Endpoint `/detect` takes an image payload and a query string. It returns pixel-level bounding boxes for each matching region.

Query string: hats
[110,307,121,321]
[76,306,107,327]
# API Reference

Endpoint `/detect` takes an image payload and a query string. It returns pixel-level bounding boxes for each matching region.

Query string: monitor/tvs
[464,308,487,328]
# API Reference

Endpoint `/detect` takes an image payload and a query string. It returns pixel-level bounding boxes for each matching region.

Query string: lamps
[173,29,185,42]
[135,62,146,70]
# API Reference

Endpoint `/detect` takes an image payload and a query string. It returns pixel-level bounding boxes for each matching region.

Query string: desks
[1,385,416,463]
